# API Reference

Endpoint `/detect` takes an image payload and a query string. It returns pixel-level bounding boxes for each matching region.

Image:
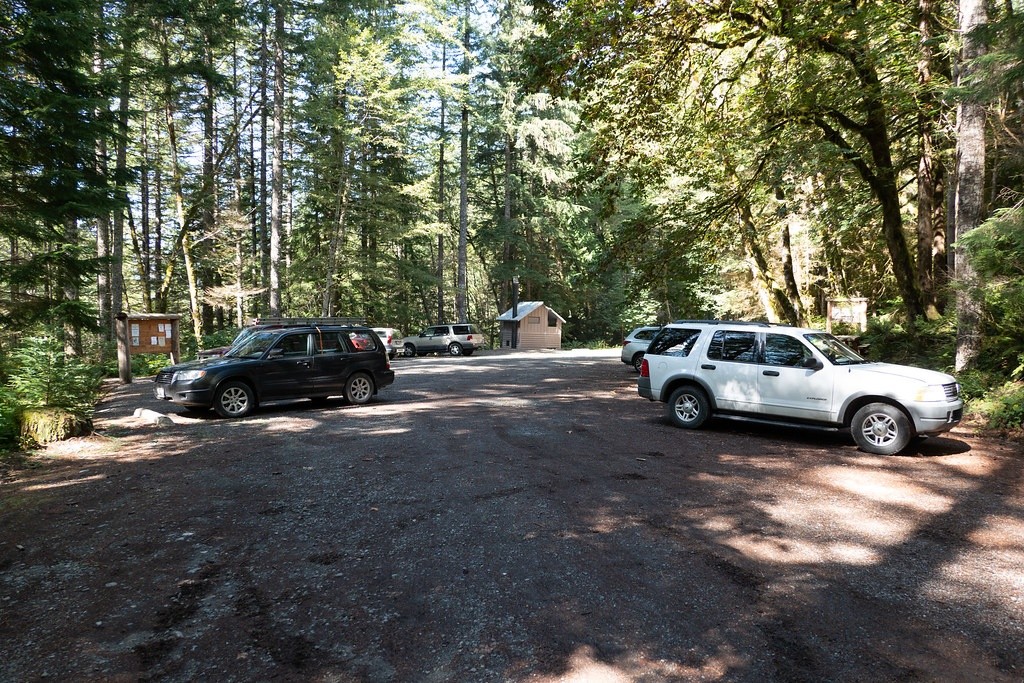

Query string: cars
[621,326,660,374]
[350,338,367,349]
[347,328,405,359]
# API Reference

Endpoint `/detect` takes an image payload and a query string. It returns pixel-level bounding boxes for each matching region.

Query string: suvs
[152,316,395,418]
[404,324,485,356]
[637,320,966,456]
